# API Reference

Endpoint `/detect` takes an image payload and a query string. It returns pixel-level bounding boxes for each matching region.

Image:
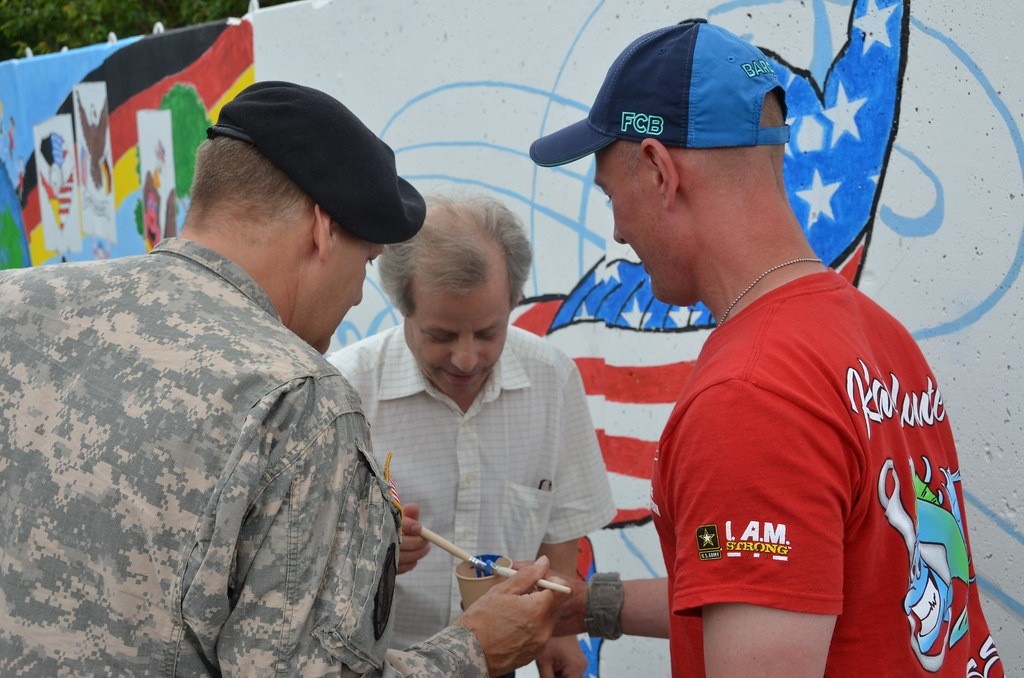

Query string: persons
[1,81,567,678]
[513,19,1007,677]
[327,195,616,678]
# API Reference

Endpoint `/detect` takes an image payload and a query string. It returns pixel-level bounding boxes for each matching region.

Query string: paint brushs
[420,526,572,597]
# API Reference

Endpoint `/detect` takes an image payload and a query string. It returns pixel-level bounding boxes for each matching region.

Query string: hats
[529,20,792,165]
[206,81,427,243]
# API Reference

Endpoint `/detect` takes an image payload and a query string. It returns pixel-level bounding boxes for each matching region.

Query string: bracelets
[584,571,625,640]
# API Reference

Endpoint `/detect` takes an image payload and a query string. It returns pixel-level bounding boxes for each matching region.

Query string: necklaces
[716,258,821,328]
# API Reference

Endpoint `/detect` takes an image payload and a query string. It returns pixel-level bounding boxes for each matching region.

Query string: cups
[455,554,513,611]
